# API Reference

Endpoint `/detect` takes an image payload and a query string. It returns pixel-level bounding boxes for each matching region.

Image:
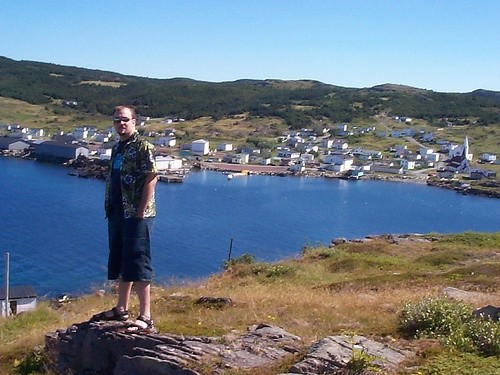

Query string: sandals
[125,316,157,334]
[90,307,130,322]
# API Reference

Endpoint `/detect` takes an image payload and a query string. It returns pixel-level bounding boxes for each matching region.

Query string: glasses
[113,117,132,123]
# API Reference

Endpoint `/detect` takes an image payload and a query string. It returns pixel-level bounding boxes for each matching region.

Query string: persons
[92,104,160,334]
